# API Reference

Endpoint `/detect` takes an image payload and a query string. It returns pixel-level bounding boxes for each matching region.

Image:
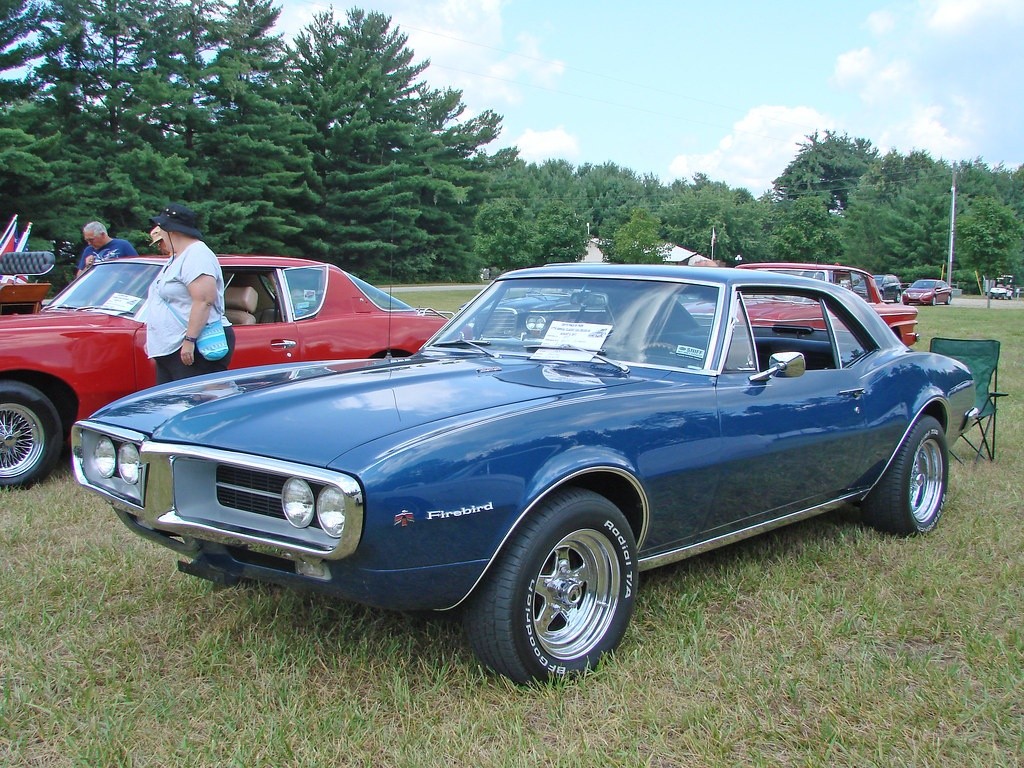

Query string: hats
[148,205,203,237]
[149,226,163,246]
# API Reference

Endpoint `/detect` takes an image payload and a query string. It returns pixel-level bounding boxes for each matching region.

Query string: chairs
[225,286,258,324]
[930,337,1001,467]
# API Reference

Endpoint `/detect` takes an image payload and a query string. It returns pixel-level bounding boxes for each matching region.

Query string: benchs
[755,336,837,371]
[0,251,55,284]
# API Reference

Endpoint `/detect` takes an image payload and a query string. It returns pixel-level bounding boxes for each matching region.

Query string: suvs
[853,274,902,304]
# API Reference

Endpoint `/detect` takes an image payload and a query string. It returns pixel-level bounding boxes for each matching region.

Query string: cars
[681,263,920,348]
[0,254,473,489]
[458,264,610,341]
[902,278,953,305]
[72,263,978,677]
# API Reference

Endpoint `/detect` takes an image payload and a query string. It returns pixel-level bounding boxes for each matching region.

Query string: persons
[145,205,235,385]
[76,221,140,278]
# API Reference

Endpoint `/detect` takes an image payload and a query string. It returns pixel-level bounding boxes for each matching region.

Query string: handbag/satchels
[195,319,229,361]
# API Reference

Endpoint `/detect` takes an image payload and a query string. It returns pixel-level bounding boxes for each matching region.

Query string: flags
[0,215,29,284]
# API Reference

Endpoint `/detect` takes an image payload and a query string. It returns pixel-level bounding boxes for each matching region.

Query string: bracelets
[185,335,196,342]
